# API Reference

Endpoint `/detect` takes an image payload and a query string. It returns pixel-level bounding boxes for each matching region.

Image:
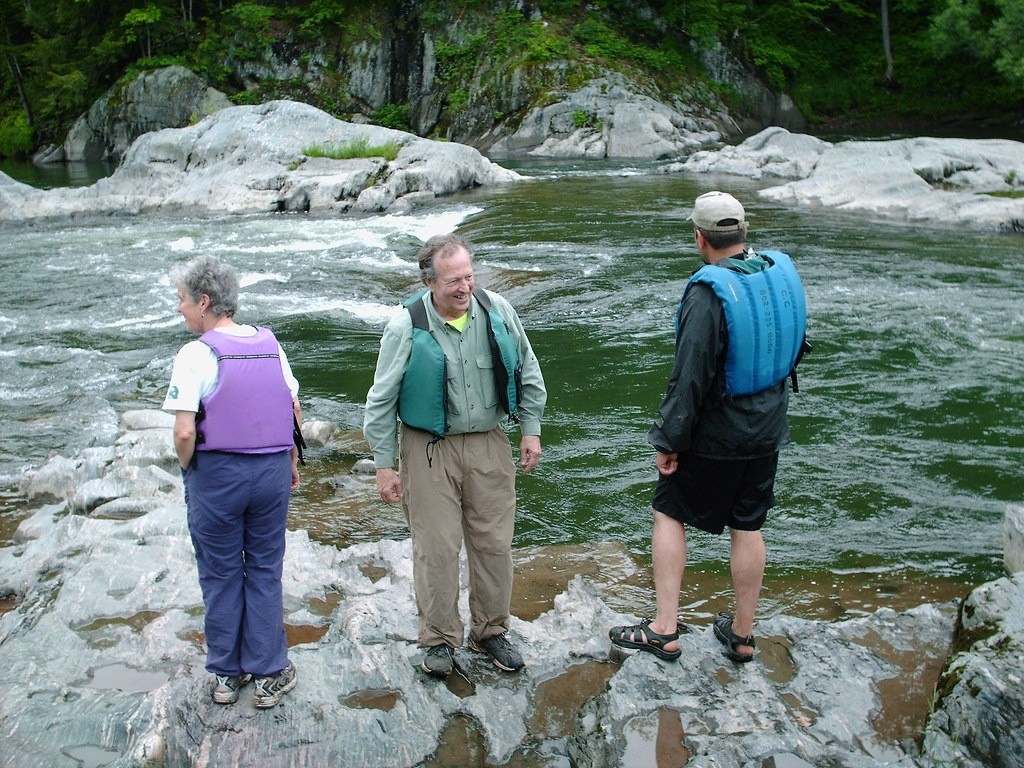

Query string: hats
[686,191,750,232]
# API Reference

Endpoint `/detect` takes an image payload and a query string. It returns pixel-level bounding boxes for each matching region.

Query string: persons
[609,192,807,662]
[364,234,547,676]
[161,256,304,707]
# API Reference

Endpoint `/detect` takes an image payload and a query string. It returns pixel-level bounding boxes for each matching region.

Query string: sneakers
[467,631,525,672]
[420,643,454,678]
[254,659,296,708]
[214,674,252,703]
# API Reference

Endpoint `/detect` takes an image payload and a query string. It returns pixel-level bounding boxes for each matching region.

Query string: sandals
[608,618,682,659]
[714,611,755,662]
